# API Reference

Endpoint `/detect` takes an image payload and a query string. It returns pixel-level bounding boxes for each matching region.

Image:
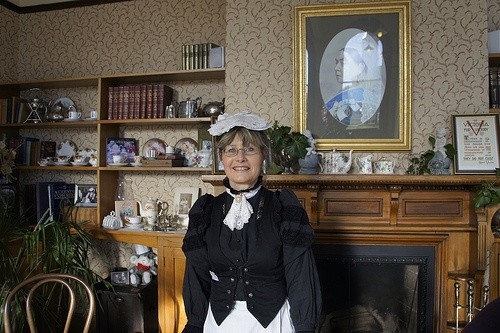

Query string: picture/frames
[292,0,412,152]
[74,183,98,206]
[173,186,200,227]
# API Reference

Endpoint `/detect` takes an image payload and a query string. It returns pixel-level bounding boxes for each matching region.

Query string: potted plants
[267,122,308,174]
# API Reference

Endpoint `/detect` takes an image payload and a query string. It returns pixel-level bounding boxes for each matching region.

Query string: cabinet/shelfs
[0,67,227,227]
[161,246,188,333]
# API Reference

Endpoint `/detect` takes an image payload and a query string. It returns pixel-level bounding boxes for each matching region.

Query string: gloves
[181,320,204,333]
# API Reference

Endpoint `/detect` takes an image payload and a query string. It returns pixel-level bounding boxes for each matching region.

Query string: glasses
[221,145,262,157]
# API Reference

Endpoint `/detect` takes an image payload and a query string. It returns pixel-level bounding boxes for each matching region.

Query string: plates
[51,97,74,110]
[109,163,143,167]
[83,118,96,121]
[140,137,167,158]
[125,223,146,228]
[174,137,199,167]
[63,118,82,122]
[54,139,96,166]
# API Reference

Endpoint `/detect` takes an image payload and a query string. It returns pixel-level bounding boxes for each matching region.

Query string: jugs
[354,154,373,173]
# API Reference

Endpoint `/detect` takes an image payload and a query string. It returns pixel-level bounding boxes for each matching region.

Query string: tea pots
[317,147,353,174]
[137,197,164,224]
[202,101,225,115]
[177,97,202,118]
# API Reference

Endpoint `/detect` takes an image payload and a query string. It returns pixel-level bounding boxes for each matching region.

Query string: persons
[180,196,191,212]
[180,113,322,333]
[86,188,96,202]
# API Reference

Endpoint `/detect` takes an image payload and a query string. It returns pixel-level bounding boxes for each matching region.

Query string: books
[0,96,28,124]
[34,182,74,223]
[2,133,56,166]
[108,84,173,120]
[182,43,220,70]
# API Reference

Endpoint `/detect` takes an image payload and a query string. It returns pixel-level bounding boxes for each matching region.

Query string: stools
[446,269,474,333]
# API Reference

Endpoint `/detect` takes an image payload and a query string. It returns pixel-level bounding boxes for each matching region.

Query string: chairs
[5,273,95,333]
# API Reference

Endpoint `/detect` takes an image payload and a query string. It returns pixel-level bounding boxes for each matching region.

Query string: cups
[91,111,97,118]
[374,157,395,173]
[194,152,211,167]
[124,216,142,224]
[112,155,143,163]
[38,155,97,167]
[68,111,82,119]
